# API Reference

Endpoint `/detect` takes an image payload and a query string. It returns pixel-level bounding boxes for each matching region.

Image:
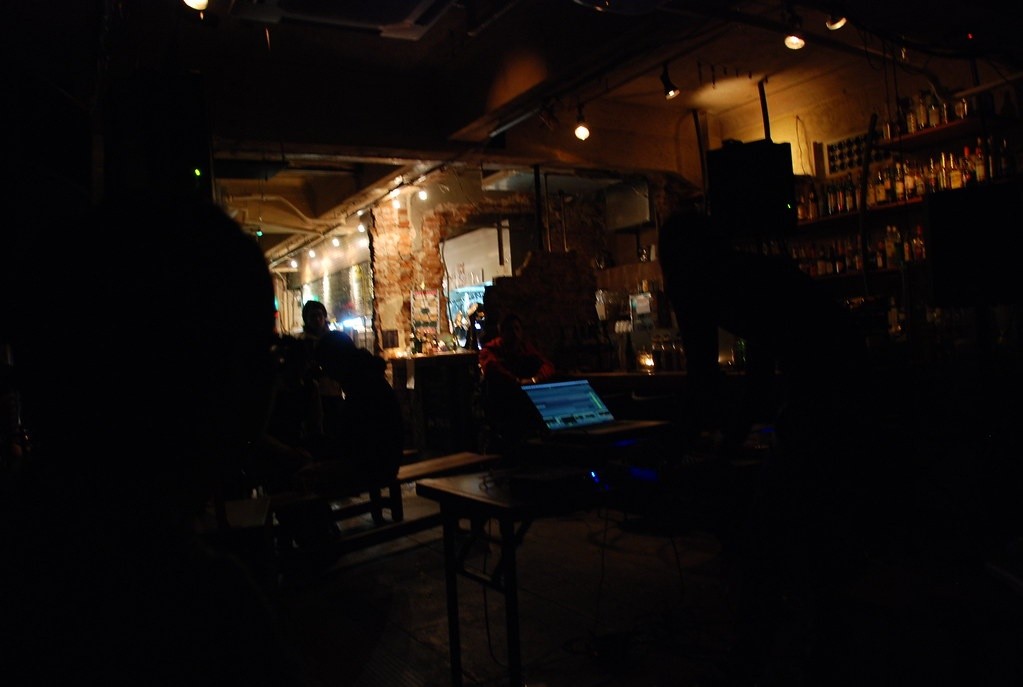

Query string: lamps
[660,65,680,100]
[572,106,594,142]
[822,0,847,30]
[781,0,806,51]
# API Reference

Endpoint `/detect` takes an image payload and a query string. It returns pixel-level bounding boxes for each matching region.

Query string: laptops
[520,378,669,445]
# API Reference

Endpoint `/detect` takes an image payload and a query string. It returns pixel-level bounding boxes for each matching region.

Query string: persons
[259,301,403,480]
[478,314,556,408]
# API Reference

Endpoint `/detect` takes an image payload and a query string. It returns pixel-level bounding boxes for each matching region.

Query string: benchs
[314,450,422,536]
[278,450,503,578]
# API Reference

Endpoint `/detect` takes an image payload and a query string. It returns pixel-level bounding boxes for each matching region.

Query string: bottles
[595,286,626,322]
[797,183,818,220]
[907,92,968,133]
[827,171,862,214]
[652,332,682,372]
[875,137,1010,205]
[630,280,657,326]
[876,225,926,269]
[791,235,863,278]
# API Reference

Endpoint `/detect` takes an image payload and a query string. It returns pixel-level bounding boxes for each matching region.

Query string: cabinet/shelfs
[795,117,1002,279]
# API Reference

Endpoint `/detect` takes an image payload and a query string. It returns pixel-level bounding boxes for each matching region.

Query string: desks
[417,453,760,687]
